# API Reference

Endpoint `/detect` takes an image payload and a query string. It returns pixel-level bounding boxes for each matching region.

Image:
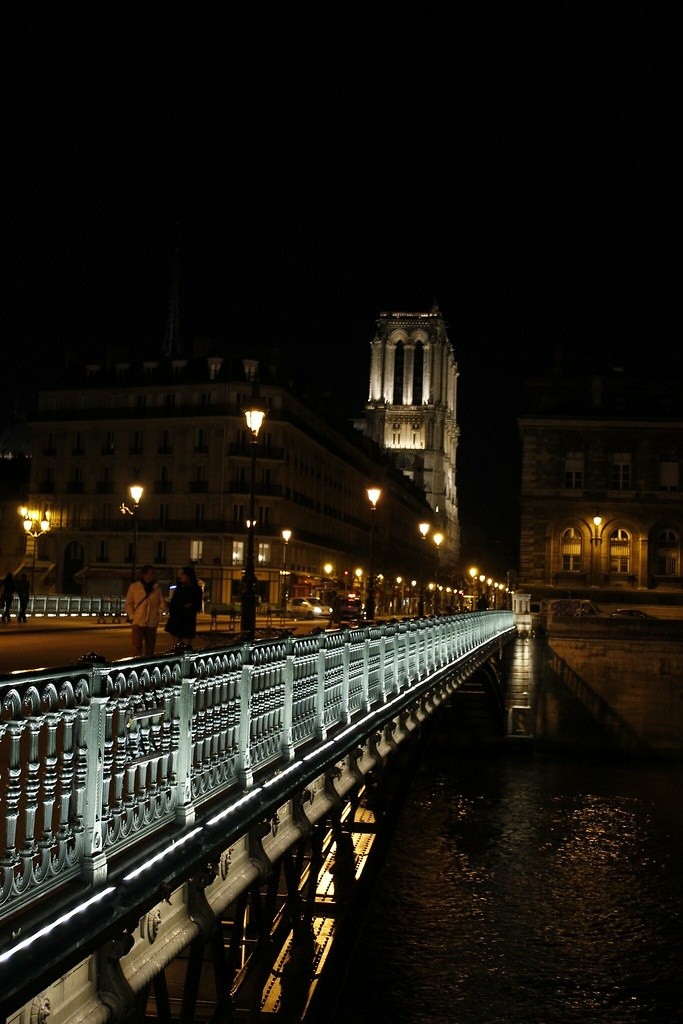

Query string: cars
[609,607,657,620]
[287,597,333,620]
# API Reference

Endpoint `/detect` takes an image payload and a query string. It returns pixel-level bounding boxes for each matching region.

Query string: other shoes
[2,619,5,622]
[7,619,10,621]
[18,619,21,623]
[23,620,27,622]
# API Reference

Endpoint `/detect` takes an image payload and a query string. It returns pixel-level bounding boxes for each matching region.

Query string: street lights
[486,578,492,609]
[417,522,430,617]
[470,568,476,610]
[365,488,382,619]
[119,485,145,581]
[494,582,498,610]
[479,575,486,598]
[239,405,267,646]
[433,533,444,616]
[280,529,291,609]
[23,520,51,594]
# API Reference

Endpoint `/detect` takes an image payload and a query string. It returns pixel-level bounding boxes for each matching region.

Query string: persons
[164,566,202,656]
[1,572,14,623]
[365,591,375,625]
[126,565,165,658]
[475,593,490,611]
[450,588,464,614]
[328,590,341,626]
[15,573,29,623]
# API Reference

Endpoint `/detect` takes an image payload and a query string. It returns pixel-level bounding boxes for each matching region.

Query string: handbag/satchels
[0,599,5,608]
